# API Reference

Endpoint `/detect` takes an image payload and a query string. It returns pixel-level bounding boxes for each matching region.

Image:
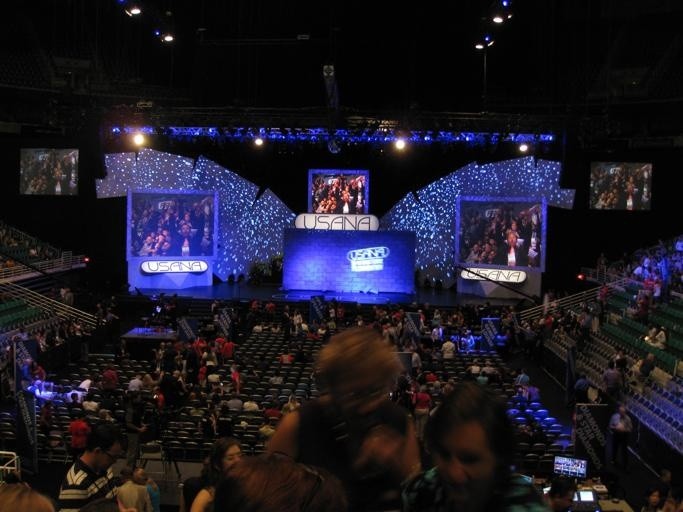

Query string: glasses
[105,452,120,461]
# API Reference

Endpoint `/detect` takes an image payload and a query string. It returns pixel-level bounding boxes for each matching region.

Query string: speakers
[79,141,107,178]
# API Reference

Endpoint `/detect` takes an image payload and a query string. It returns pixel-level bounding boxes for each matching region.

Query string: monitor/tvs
[553,455,587,480]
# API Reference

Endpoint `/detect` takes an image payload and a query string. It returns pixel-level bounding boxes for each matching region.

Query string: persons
[589,161,652,210]
[131,193,212,256]
[460,201,539,267]
[0,226,683,512]
[20,149,77,195]
[312,174,364,214]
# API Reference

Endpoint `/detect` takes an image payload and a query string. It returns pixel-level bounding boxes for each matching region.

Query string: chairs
[0,225,683,512]
